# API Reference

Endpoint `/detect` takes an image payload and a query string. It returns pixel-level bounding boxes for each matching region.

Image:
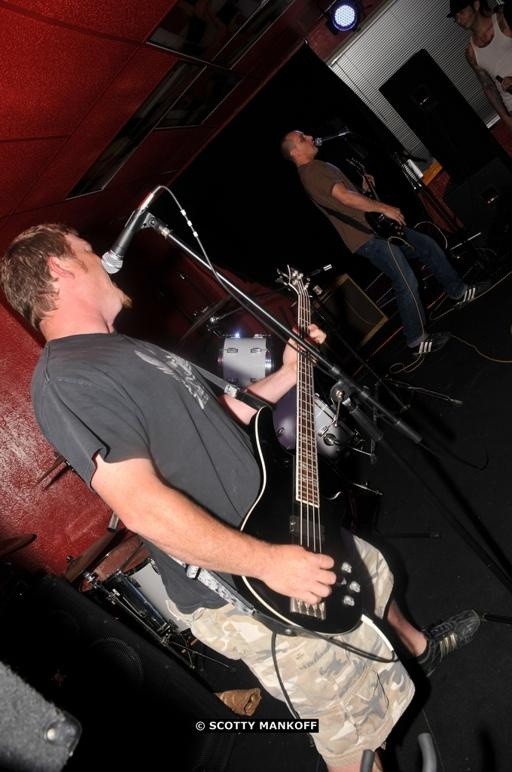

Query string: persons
[1,223,486,771]
[274,125,487,368]
[443,0,510,126]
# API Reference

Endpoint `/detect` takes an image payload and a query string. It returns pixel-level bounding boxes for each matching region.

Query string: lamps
[330,2,360,32]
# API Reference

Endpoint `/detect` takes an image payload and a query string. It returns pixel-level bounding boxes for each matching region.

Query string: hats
[446,0,481,18]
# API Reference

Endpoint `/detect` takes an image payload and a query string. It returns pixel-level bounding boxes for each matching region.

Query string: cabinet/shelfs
[317,273,389,350]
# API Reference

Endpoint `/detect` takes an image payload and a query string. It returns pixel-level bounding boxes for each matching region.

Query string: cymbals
[181,294,233,340]
[34,454,64,488]
[1,532,37,556]
[53,527,122,600]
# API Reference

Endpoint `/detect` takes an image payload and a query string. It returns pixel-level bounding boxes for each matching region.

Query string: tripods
[208,264,464,464]
[124,211,512,624]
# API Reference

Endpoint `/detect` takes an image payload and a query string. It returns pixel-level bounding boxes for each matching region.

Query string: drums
[124,542,191,633]
[217,333,273,391]
[272,385,360,473]
[81,533,165,637]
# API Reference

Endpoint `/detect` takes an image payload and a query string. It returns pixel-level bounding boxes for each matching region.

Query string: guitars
[231,265,375,636]
[347,158,406,246]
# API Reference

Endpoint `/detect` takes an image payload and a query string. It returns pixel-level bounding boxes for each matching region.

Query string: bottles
[496,75,512,95]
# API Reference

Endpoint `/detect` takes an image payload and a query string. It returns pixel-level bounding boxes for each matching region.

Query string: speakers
[378,49,500,186]
[0,573,240,772]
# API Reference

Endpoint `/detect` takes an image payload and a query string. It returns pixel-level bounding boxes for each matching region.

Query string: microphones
[101,192,157,274]
[314,130,352,147]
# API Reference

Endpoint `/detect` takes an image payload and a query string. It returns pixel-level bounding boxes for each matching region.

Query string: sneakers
[447,279,494,307]
[406,330,452,355]
[411,606,482,680]
